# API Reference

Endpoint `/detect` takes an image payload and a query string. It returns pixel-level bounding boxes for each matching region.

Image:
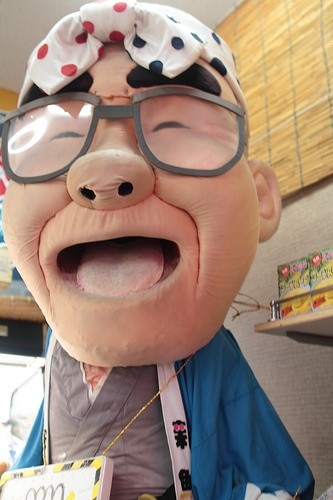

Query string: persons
[1,0,318,499]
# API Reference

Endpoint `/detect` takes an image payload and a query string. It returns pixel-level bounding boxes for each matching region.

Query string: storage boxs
[277,246,333,319]
